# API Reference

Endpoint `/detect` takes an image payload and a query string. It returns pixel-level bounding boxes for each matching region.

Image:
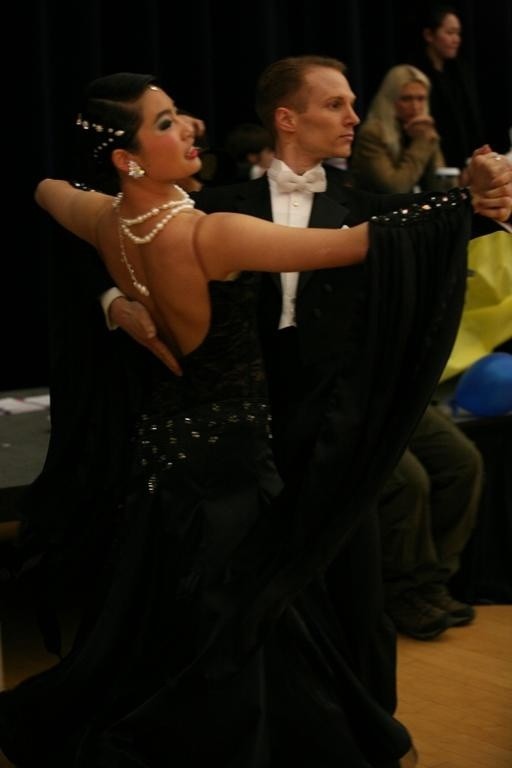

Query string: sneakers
[386,589,449,641]
[416,581,475,627]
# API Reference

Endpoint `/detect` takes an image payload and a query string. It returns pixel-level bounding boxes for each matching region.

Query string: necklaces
[106,183,197,298]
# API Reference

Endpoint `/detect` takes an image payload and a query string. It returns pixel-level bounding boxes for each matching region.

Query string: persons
[349,62,495,198]
[0,68,512,768]
[88,54,512,717]
[414,8,495,166]
[377,404,487,643]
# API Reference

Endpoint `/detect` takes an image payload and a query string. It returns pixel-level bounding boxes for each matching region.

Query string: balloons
[450,351,512,417]
